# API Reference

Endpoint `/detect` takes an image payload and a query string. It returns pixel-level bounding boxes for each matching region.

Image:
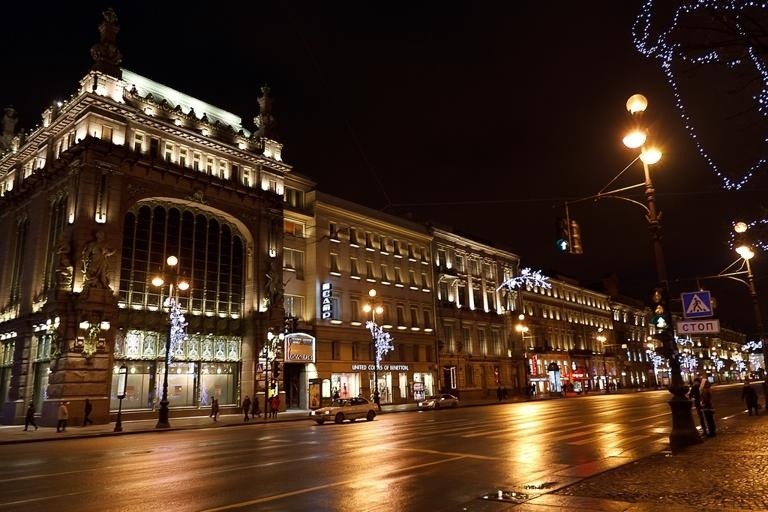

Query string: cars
[308,397,378,425]
[417,394,458,410]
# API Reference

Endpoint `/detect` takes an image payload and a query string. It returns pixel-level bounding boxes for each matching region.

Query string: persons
[688,377,716,437]
[80,398,93,427]
[610,383,615,391]
[334,391,340,400]
[57,402,69,432]
[22,400,38,431]
[741,380,759,416]
[210,395,280,422]
[497,387,508,401]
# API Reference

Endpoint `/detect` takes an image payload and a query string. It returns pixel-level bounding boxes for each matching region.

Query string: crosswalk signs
[680,290,713,319]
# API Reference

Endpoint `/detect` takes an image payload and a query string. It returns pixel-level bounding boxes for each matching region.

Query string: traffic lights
[554,217,570,253]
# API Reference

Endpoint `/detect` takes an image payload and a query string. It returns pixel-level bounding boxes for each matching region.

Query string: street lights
[595,327,610,395]
[514,314,529,393]
[620,90,702,451]
[731,219,766,372]
[151,255,189,431]
[362,288,383,412]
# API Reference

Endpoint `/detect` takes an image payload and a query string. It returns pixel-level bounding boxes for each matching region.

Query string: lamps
[78,321,111,358]
[267,332,285,354]
